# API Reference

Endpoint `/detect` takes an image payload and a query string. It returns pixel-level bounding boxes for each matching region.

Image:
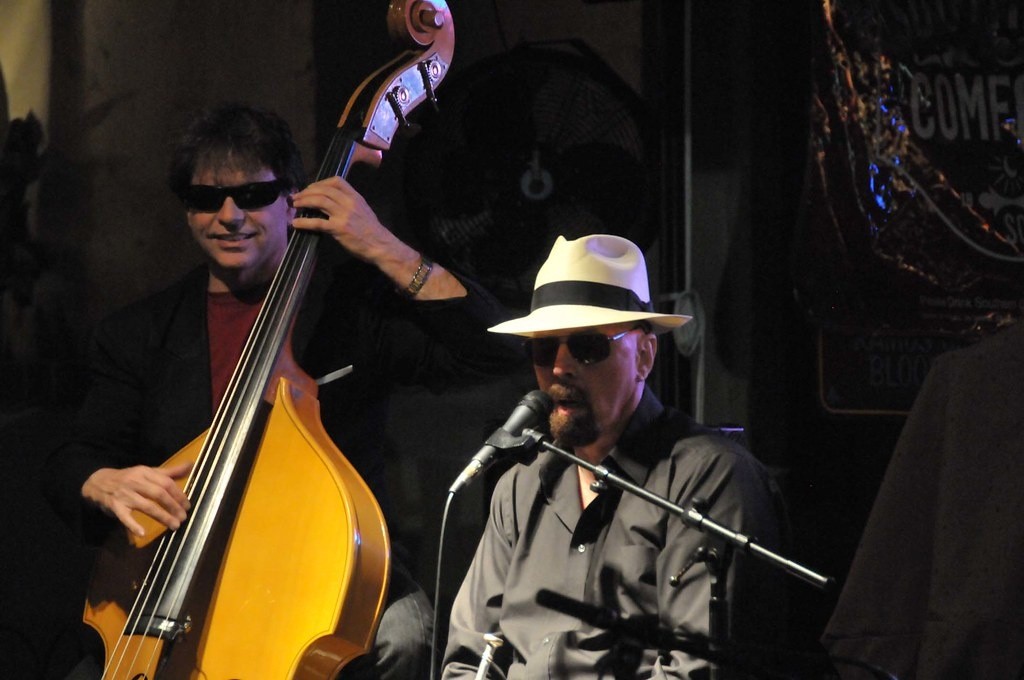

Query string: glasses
[181,173,297,213]
[523,323,649,368]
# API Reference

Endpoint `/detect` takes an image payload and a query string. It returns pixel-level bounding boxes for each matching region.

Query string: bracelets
[394,250,433,298]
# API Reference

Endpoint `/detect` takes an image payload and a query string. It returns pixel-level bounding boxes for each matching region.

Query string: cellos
[78,0,456,680]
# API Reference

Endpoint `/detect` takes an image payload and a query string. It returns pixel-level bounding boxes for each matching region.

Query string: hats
[486,232,693,338]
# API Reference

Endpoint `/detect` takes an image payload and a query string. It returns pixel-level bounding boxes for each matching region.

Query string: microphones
[448,390,554,496]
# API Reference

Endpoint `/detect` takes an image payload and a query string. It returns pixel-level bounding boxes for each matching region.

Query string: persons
[34,101,520,679]
[440,234,794,680]
